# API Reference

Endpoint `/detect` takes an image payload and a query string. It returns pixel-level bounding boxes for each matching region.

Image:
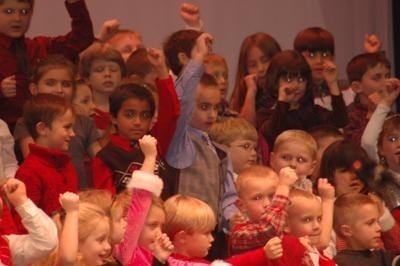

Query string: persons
[313,139,400,260]
[13,53,103,193]
[0,119,20,202]
[80,42,127,195]
[163,32,249,261]
[359,77,400,226]
[293,26,382,134]
[78,17,144,64]
[85,47,181,202]
[229,164,299,257]
[338,52,400,167]
[161,192,284,266]
[270,129,318,193]
[0,0,95,167]
[87,81,160,160]
[258,49,350,167]
[110,134,167,266]
[307,125,345,184]
[163,2,214,77]
[77,189,174,266]
[271,177,337,266]
[10,92,81,235]
[209,116,259,182]
[27,190,112,266]
[125,46,170,91]
[203,52,240,124]
[0,178,59,266]
[70,76,97,122]
[332,191,400,266]
[229,32,283,132]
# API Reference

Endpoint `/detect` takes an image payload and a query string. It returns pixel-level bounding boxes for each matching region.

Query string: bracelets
[93,37,104,43]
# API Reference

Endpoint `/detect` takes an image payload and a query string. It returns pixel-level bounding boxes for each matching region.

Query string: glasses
[229,142,256,151]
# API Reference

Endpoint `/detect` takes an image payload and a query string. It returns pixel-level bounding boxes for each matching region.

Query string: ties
[11,40,29,108]
